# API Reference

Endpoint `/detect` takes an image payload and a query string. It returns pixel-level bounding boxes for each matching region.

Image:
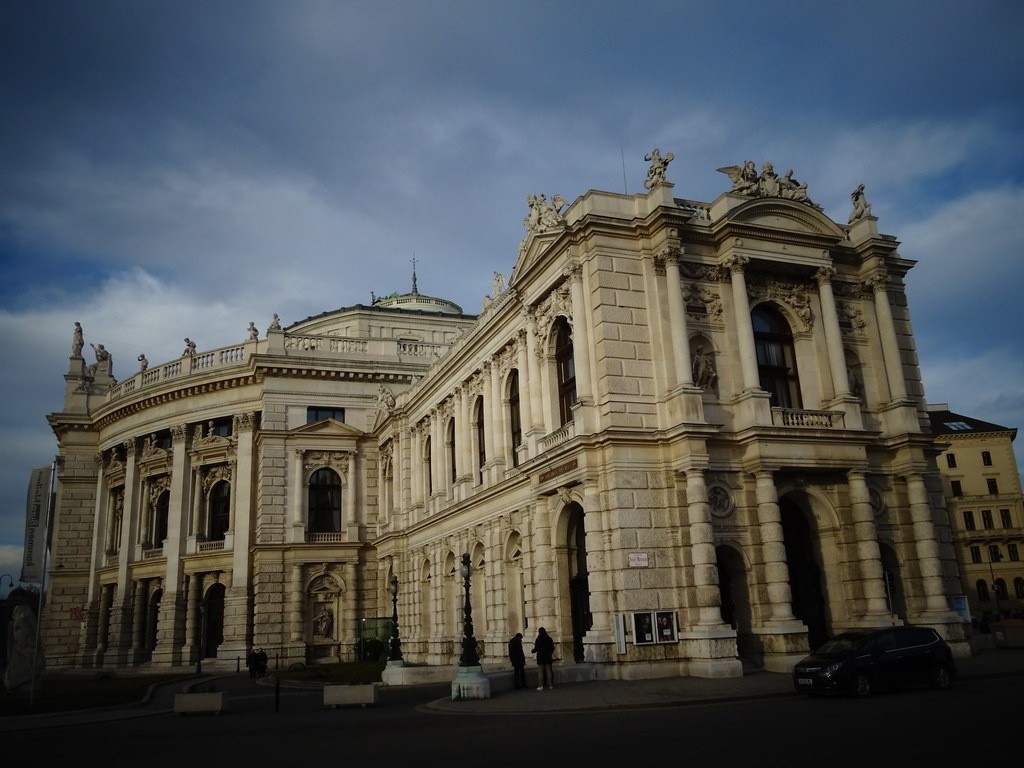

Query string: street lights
[451,552,491,701]
[381,575,404,684]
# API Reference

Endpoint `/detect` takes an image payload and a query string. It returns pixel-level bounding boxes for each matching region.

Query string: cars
[793,625,957,700]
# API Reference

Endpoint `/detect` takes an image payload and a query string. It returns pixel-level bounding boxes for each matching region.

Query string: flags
[71,607,83,621]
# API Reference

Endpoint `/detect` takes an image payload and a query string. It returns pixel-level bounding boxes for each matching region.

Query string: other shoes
[536,686,544,690]
[549,686,554,690]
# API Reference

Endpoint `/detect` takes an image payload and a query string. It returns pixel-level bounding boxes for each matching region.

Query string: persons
[727,161,821,207]
[137,354,149,370]
[90,344,105,361]
[151,433,159,449]
[206,421,215,442]
[378,383,387,401]
[312,605,329,635]
[851,184,868,211]
[509,633,530,689]
[72,322,84,356]
[246,648,267,679]
[791,289,810,325]
[530,627,555,691]
[644,148,670,179]
[245,321,259,341]
[182,338,196,356]
[107,448,120,470]
[692,345,716,389]
[267,314,281,330]
[8,606,39,684]
